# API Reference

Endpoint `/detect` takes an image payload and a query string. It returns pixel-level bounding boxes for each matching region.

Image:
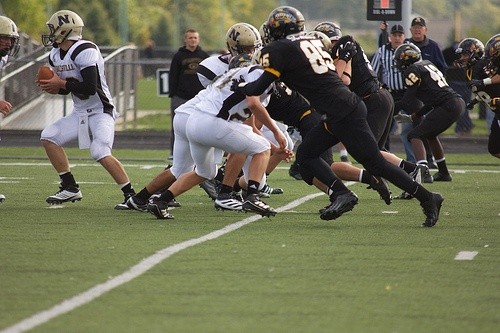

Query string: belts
[299,109,311,121]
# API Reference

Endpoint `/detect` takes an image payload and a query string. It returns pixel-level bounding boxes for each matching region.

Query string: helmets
[394,43,421,68]
[0,15,19,39]
[225,6,343,69]
[46,11,84,40]
[457,38,484,65]
[484,34,500,59]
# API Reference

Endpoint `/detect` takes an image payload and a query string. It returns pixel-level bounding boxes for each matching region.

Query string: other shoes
[0,193,5,203]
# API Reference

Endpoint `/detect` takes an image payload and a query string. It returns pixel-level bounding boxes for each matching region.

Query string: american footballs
[38,66,60,94]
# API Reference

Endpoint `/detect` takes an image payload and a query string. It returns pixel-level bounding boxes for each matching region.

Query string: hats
[391,24,404,34]
[411,17,425,26]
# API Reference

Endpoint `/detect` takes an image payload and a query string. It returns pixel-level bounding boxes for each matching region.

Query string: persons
[168,29,209,159]
[127,17,500,219]
[0,16,20,203]
[229,6,445,228]
[34,9,137,210]
[394,42,466,183]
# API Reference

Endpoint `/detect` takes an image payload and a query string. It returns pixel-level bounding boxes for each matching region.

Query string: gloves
[339,41,357,62]
[230,76,245,93]
[469,78,485,87]
[394,114,413,124]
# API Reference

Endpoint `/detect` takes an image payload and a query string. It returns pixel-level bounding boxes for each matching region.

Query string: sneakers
[147,197,175,219]
[366,171,392,205]
[46,186,83,205]
[420,193,444,227]
[199,168,283,217]
[115,194,136,210]
[320,189,358,221]
[404,163,428,198]
[127,196,150,212]
[424,176,433,183]
[433,172,452,182]
[149,193,181,207]
